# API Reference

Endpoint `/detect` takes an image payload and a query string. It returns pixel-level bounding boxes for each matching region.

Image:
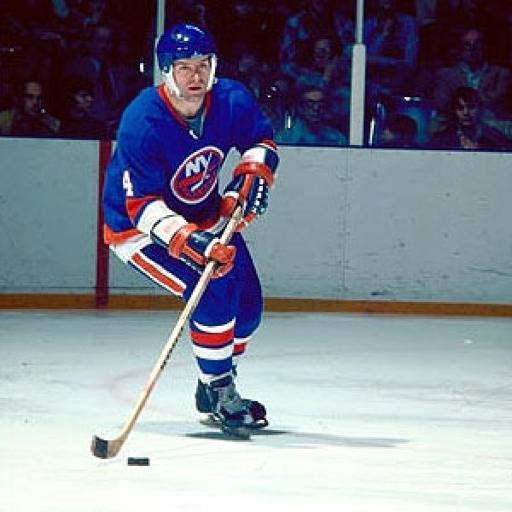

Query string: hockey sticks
[90,206,242,458]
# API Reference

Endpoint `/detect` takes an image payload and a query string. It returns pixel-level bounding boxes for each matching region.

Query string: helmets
[156,22,220,100]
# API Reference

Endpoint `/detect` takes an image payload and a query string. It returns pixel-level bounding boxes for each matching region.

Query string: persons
[0,1,512,152]
[100,24,282,427]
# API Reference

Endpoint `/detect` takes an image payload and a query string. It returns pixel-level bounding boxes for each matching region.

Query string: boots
[195,356,267,428]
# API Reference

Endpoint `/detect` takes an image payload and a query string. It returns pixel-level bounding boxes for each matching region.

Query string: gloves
[220,161,274,225]
[167,223,237,278]
